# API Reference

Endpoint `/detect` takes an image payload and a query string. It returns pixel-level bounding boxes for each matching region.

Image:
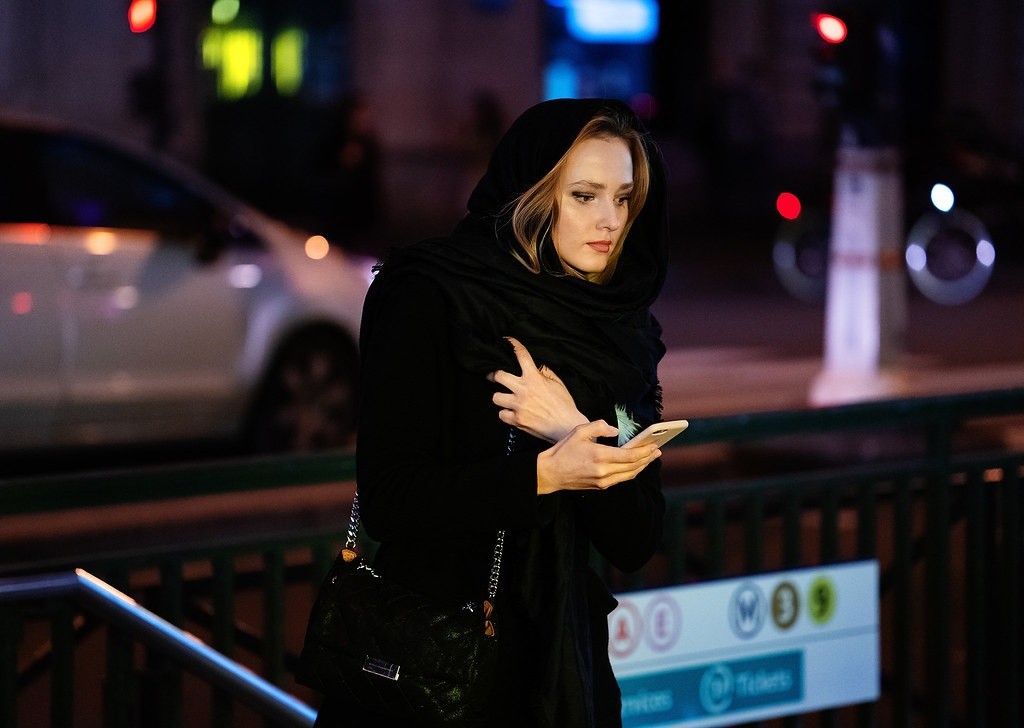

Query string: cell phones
[622,420,689,449]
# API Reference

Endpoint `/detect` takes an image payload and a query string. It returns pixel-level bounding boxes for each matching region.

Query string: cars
[753,113,1024,307]
[0,110,385,477]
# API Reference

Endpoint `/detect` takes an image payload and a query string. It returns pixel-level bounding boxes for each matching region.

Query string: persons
[351,97,671,728]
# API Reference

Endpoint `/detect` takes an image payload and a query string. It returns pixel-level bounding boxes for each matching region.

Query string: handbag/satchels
[298,550,503,727]
